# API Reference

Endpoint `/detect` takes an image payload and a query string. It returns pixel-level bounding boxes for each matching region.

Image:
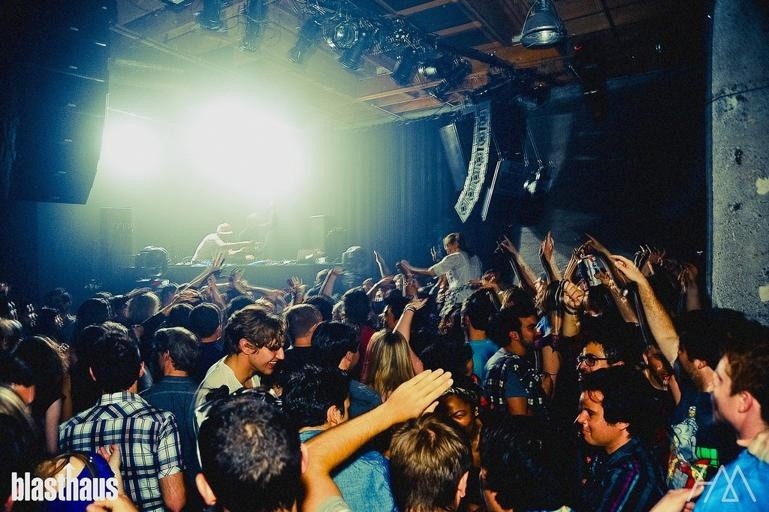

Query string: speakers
[307,215,336,256]
[11,0,117,203]
[98,207,132,270]
[439,100,529,225]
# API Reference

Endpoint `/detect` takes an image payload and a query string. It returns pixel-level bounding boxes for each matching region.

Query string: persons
[191,223,252,265]
[0,233,769,512]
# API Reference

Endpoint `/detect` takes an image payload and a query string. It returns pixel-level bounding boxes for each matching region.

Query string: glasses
[576,354,604,364]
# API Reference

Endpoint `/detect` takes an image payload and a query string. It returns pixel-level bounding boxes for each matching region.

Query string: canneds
[576,253,603,291]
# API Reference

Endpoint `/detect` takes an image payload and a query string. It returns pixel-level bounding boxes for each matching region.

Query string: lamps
[520,0,567,52]
[578,68,613,122]
[561,1,705,78]
[154,1,562,111]
